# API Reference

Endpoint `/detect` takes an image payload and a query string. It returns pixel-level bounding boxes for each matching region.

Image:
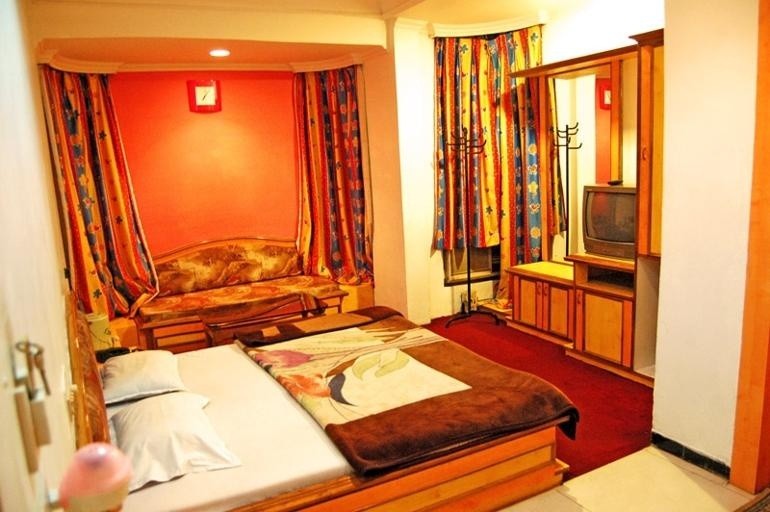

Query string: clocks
[185,79,223,114]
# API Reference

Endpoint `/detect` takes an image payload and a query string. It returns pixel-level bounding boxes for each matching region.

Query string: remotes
[608,179,622,185]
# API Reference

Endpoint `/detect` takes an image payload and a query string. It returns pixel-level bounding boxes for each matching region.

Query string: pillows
[97,349,187,405]
[105,389,245,497]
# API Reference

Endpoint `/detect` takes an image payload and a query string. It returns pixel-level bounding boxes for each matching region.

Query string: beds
[64,284,573,512]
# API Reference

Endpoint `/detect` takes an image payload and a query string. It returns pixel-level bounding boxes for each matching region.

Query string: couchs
[104,237,376,354]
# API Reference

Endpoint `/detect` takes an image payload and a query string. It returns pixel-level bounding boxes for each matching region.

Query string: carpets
[419,309,655,483]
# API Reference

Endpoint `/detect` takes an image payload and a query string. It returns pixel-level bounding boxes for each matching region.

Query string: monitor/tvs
[582,185,636,263]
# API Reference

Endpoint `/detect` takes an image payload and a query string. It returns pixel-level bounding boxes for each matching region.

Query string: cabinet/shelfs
[503,253,664,389]
[628,27,663,259]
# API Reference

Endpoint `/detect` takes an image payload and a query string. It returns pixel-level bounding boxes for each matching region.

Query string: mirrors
[543,64,611,267]
